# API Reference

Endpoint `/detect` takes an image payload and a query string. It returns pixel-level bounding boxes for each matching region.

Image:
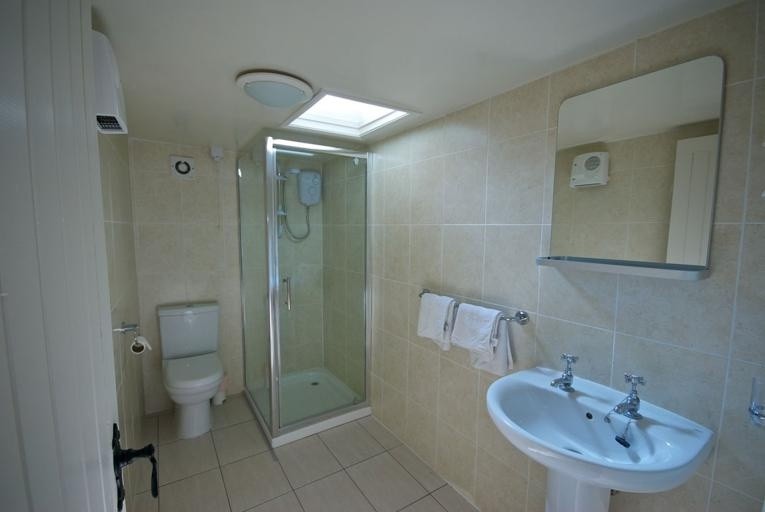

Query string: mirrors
[535,55,727,280]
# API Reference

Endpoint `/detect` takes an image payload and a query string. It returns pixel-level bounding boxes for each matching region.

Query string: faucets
[547,352,580,393]
[611,374,648,420]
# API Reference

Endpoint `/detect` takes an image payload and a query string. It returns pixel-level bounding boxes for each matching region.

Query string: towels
[450,302,514,369]
[417,293,456,352]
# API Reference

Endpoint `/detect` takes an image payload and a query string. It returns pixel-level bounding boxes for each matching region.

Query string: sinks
[486,366,715,491]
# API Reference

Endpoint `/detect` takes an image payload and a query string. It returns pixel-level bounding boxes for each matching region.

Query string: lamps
[234,71,314,108]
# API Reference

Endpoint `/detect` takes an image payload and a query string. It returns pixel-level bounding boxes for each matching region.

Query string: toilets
[154,301,224,440]
